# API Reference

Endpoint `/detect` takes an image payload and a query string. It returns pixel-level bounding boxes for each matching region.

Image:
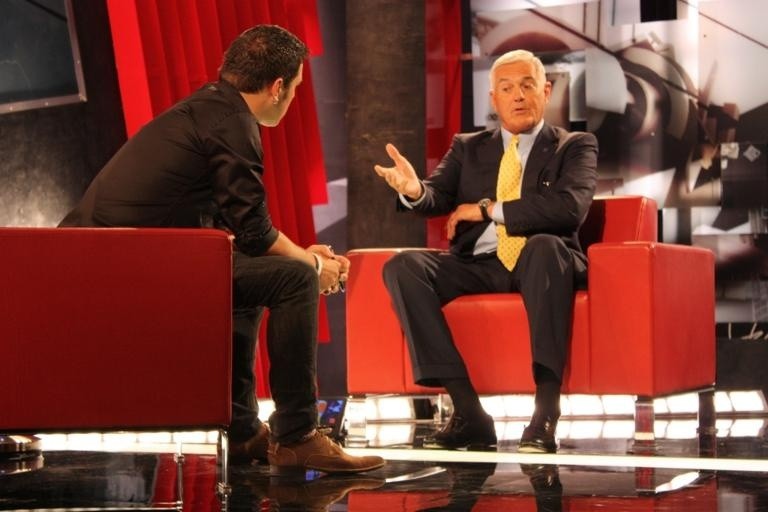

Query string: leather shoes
[516,408,560,453]
[228,424,269,465]
[433,462,497,512]
[231,475,386,512]
[274,431,385,472]
[520,463,563,512]
[422,414,498,447]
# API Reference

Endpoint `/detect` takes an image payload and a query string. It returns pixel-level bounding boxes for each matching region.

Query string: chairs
[329,192,720,443]
[1,219,239,510]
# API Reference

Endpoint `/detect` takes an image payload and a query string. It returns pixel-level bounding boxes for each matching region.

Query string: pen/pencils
[328,245,344,290]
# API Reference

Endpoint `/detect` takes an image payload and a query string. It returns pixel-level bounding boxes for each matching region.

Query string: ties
[496,135,527,274]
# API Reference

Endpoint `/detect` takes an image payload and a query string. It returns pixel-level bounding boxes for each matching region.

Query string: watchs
[478,198,492,221]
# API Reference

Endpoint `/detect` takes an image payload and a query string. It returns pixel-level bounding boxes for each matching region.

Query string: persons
[224,462,565,512]
[375,49,598,453]
[57,23,385,474]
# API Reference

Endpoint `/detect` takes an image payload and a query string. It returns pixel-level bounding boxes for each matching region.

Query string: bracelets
[314,253,322,276]
